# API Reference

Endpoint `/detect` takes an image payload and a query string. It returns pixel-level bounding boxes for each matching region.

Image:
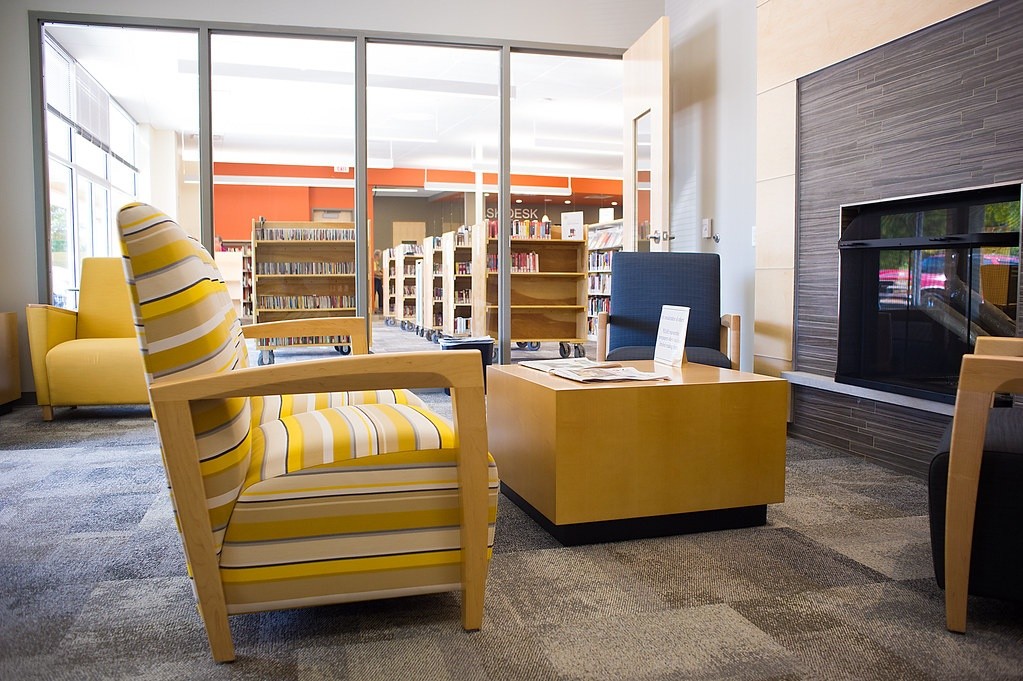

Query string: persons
[373,249,383,312]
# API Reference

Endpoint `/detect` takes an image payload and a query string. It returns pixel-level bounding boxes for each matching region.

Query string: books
[222,227,356,347]
[389,220,624,334]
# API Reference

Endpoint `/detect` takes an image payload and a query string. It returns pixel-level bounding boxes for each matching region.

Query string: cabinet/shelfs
[251,218,373,366]
[220,240,252,321]
[588,219,624,342]
[383,224,589,358]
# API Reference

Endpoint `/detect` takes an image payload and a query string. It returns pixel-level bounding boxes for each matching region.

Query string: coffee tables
[488,358,789,546]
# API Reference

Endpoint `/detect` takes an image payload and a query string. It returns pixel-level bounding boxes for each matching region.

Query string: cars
[879,253,1019,309]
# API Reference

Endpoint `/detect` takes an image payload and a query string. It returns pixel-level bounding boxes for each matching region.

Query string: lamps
[181,132,393,170]
[424,169,571,196]
[182,163,355,188]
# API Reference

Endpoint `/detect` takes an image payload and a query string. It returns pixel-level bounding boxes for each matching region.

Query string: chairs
[928,336,1023,634]
[26,256,150,421]
[597,252,740,370]
[0,311,21,415]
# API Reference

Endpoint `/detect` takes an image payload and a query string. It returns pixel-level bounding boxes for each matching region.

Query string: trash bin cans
[437,336,496,397]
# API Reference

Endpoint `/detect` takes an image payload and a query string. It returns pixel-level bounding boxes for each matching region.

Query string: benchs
[116,203,499,665]
